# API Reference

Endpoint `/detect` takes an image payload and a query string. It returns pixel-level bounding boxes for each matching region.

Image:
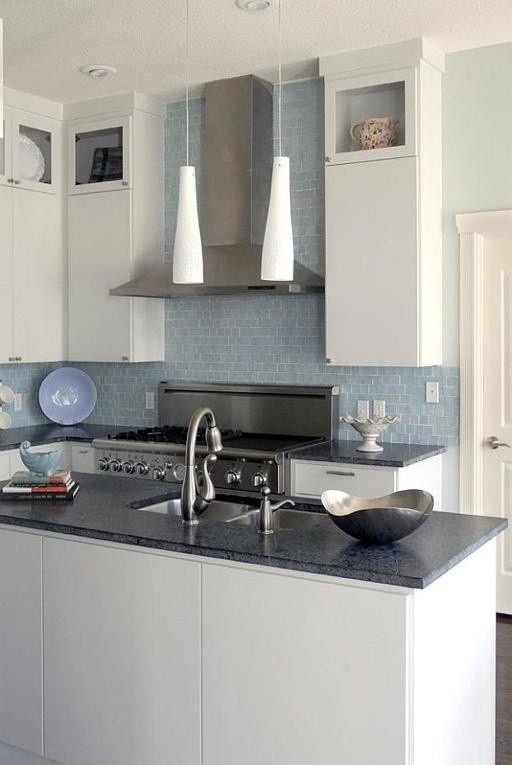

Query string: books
[0,470,81,501]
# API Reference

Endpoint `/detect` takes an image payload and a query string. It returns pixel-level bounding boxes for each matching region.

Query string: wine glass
[341,414,400,454]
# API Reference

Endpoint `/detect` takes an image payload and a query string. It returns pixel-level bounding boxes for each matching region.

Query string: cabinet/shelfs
[0,86,65,364]
[319,36,444,368]
[291,454,444,512]
[64,91,166,363]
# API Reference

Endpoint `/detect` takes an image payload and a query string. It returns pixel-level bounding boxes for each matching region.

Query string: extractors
[108,73,325,298]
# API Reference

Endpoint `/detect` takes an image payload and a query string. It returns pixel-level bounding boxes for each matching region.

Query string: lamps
[261,0,294,282]
[172,0,204,284]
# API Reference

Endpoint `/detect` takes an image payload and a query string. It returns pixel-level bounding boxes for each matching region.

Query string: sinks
[134,498,259,523]
[228,510,330,529]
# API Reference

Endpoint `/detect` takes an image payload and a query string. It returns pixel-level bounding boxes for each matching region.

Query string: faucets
[257,487,295,535]
[179,406,223,528]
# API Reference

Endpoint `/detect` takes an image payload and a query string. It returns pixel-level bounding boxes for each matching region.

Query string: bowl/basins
[319,486,435,545]
[19,439,65,475]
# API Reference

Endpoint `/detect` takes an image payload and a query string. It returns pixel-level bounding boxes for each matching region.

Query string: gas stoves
[92,423,325,495]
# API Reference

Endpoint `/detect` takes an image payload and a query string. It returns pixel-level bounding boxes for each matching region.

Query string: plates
[38,366,98,428]
[12,134,46,183]
